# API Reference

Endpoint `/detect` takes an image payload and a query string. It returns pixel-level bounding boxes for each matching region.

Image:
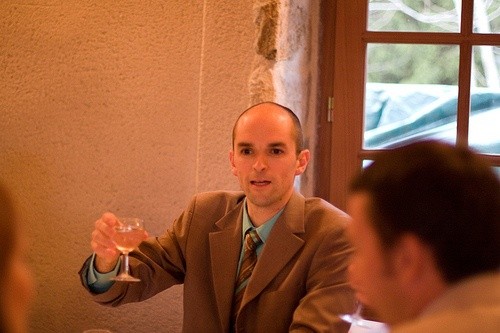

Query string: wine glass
[109,218,142,282]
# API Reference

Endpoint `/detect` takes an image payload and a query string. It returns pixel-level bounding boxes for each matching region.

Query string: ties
[228,229,263,332]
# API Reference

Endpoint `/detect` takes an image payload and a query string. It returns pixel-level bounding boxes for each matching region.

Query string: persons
[0,185,36,333]
[338,139,500,333]
[79,102,359,333]
